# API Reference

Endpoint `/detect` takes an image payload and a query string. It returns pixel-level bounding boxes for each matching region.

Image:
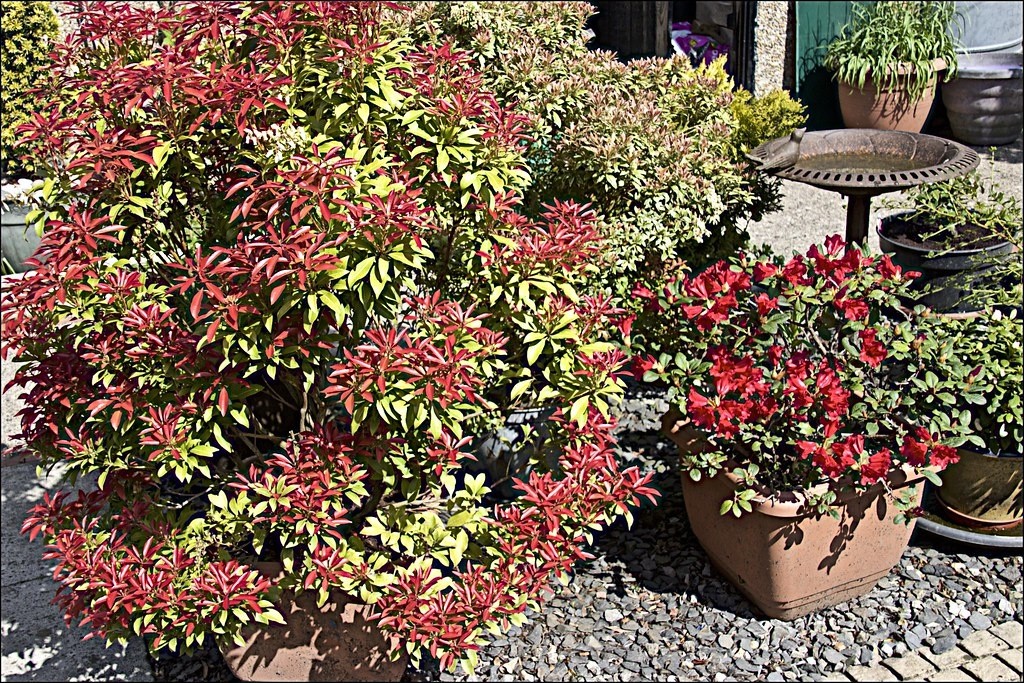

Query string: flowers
[669,22,731,70]
[629,233,961,540]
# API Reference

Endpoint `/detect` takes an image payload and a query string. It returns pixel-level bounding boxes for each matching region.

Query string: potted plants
[0,0,1024,683]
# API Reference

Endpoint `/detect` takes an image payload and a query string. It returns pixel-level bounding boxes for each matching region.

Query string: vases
[938,51,1024,145]
[661,406,947,623]
